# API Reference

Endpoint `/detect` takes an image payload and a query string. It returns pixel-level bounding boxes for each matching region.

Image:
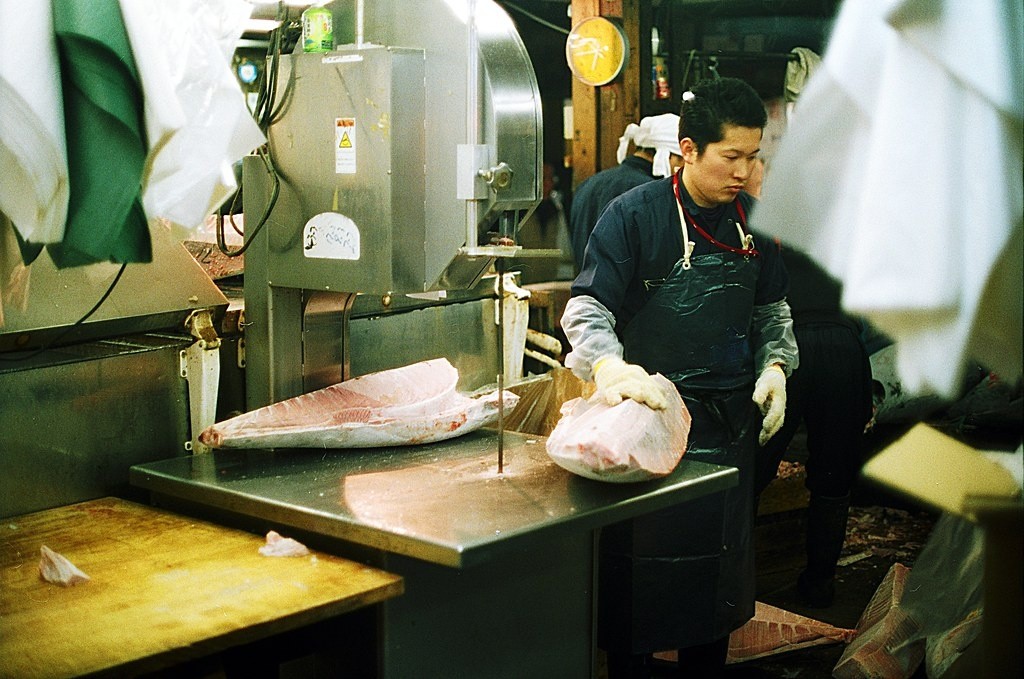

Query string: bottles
[652,57,673,105]
[301,2,338,53]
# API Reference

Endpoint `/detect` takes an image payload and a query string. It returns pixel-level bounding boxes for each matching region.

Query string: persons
[569,98,685,280]
[559,75,799,679]
[754,246,872,609]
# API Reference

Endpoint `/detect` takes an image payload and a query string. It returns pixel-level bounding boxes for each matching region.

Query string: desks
[0,494,406,679]
[129,427,740,679]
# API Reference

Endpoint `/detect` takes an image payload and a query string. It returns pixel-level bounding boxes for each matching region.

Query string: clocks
[566,15,631,87]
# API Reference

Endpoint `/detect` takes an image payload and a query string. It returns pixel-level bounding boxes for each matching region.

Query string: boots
[798,493,851,609]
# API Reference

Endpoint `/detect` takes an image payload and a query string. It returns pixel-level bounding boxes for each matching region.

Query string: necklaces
[673,169,757,255]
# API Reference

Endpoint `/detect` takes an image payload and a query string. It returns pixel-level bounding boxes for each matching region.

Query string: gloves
[593,355,671,410]
[753,366,785,446]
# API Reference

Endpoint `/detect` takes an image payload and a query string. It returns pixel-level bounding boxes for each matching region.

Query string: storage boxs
[859,421,1024,679]
